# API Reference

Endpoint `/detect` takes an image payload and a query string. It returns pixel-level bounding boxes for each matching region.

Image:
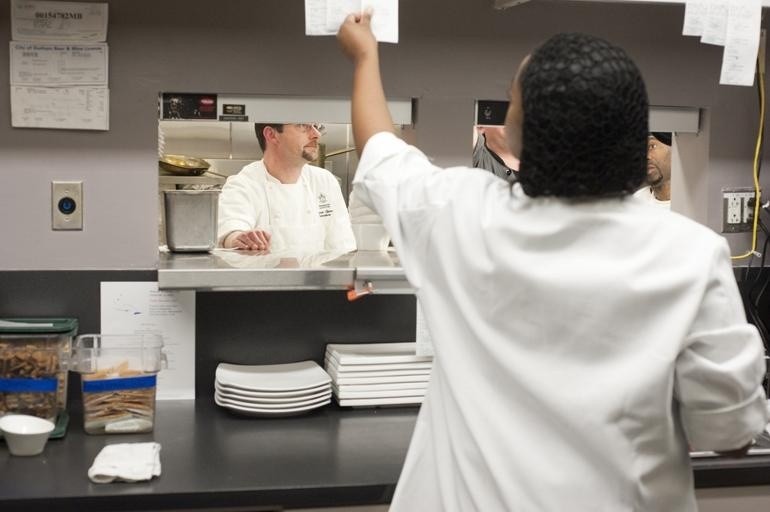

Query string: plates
[324,342,433,408]
[213,360,332,416]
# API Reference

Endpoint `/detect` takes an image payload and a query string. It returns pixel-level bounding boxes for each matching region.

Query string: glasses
[283,123,327,134]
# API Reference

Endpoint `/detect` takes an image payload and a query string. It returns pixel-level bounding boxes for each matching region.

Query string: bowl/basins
[349,191,391,250]
[0,413,55,456]
[350,250,394,269]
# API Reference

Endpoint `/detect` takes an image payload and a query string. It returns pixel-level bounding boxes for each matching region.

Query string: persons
[218,123,357,250]
[472,126,521,183]
[632,131,671,206]
[337,7,769,512]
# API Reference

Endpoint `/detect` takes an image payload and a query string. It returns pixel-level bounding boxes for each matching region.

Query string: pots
[159,154,228,179]
[308,142,355,169]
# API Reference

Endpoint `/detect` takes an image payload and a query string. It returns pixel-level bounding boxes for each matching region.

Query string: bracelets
[222,230,244,247]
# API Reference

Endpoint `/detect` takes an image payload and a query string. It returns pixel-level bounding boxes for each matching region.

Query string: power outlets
[744,196,754,224]
[727,196,741,224]
